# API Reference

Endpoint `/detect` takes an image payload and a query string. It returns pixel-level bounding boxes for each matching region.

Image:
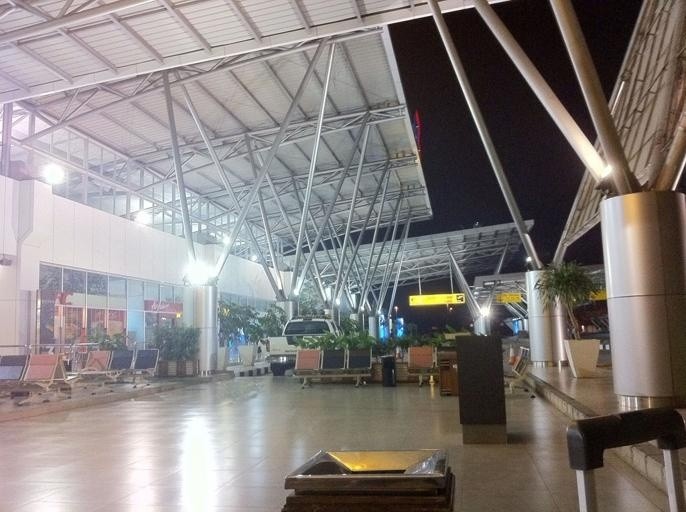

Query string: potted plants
[372,336,411,382]
[532,258,605,378]
[152,321,202,377]
[217,299,266,372]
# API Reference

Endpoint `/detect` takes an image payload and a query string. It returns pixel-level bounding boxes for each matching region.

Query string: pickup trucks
[264,318,344,377]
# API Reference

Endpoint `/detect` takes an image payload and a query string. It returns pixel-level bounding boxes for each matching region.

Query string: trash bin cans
[380,355,397,386]
[281,447,456,512]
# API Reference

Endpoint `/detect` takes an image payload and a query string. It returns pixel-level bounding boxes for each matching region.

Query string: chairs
[503,346,531,382]
[0,348,159,407]
[293,347,373,390]
